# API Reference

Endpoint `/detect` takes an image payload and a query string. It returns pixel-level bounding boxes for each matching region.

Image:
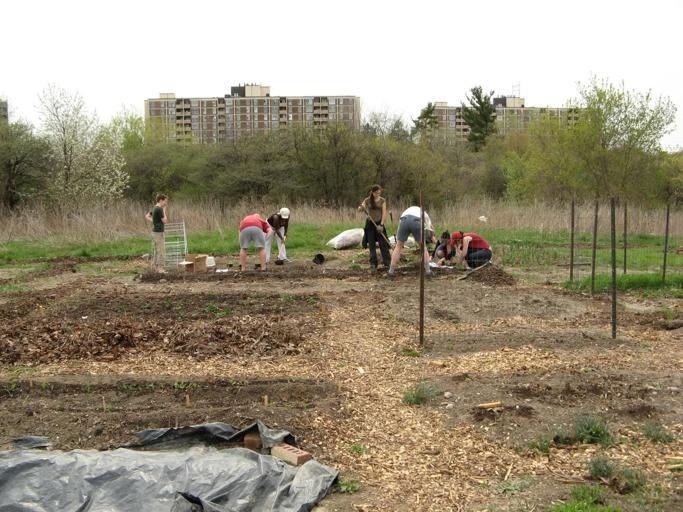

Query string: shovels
[456,261,490,281]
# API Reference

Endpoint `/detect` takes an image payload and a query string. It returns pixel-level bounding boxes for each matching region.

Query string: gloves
[377,224,384,234]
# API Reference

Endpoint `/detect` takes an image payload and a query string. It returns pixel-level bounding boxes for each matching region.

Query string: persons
[264,207,290,262]
[144,193,168,261]
[357,184,391,271]
[235,212,273,273]
[386,205,436,278]
[428,229,456,267]
[449,231,493,271]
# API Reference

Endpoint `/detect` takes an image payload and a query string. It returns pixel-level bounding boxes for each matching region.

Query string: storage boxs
[179,254,207,273]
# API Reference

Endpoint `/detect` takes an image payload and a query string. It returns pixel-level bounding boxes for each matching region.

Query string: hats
[279,207,290,220]
